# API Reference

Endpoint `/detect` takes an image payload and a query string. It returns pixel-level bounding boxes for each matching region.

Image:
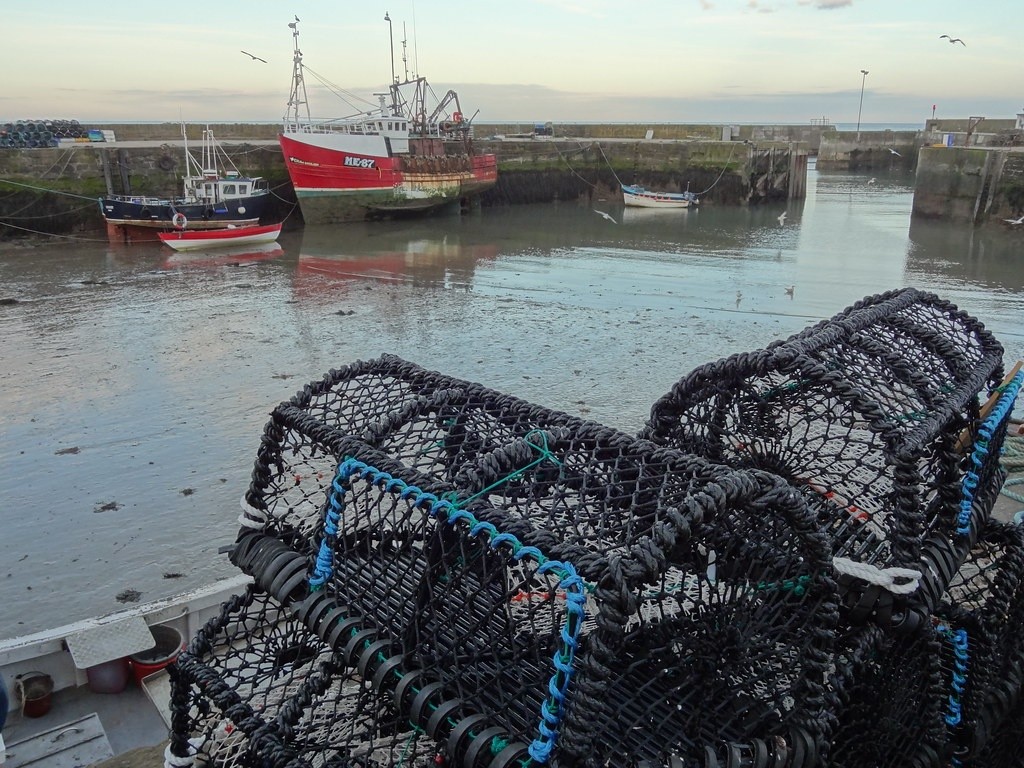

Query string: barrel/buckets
[127,624,185,687]
[16,671,55,718]
[87,657,127,695]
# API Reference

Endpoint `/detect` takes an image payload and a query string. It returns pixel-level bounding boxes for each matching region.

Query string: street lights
[857,70,870,145]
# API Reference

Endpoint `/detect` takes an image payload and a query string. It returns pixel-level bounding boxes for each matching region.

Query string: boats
[102,123,285,245]
[621,184,702,209]
[153,225,279,252]
[294,200,497,298]
[623,205,689,226]
[165,241,286,270]
[278,8,497,224]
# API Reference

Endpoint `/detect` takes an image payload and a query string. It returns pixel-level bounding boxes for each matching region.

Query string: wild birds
[940,34,966,46]
[241,50,267,64]
[1002,216,1024,224]
[785,285,796,292]
[736,290,743,299]
[593,209,617,224]
[888,148,901,157]
[868,177,876,184]
[776,210,788,226]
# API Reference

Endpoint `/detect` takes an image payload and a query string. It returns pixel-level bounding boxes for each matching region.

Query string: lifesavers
[172,212,187,230]
[201,208,215,220]
[160,156,173,169]
[168,207,181,219]
[140,208,152,219]
